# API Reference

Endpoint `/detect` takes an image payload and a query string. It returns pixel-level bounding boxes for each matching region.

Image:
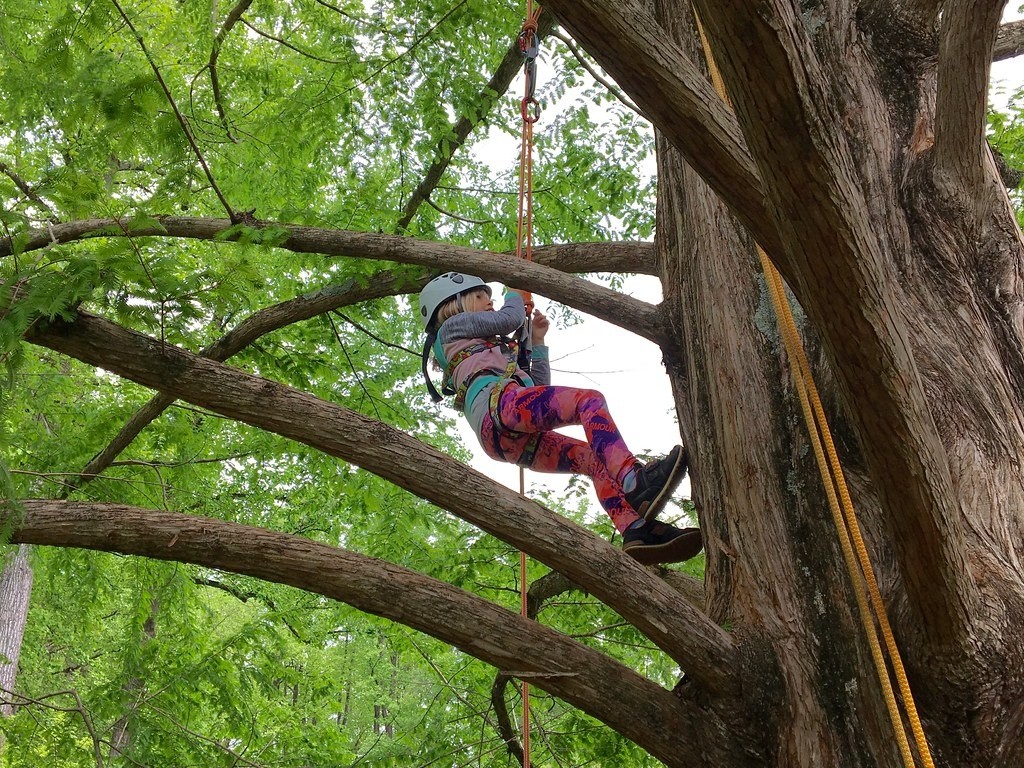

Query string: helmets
[419,271,492,334]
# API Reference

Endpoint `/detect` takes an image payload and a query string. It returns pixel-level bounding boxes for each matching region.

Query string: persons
[419,272,704,566]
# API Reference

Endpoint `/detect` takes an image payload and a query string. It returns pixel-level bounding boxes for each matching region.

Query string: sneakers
[623,520,704,566]
[625,445,687,522]
